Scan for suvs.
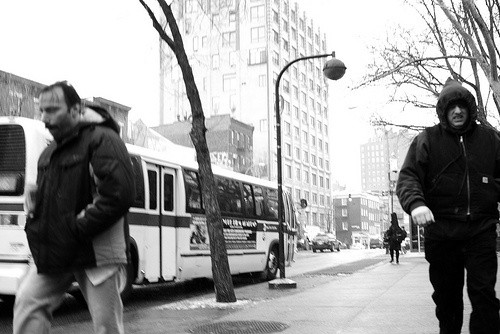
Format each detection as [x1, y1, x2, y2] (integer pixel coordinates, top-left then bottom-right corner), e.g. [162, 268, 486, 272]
[370, 238, 383, 249]
[312, 233, 342, 253]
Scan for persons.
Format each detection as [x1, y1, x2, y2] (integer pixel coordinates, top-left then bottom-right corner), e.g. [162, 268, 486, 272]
[13, 81, 136, 334]
[384, 221, 407, 264]
[396, 78, 500, 334]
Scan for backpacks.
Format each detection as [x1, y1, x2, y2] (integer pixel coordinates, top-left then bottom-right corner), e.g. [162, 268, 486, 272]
[395, 229, 405, 242]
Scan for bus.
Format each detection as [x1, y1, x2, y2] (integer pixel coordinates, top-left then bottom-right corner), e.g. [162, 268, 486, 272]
[0, 116, 308, 306]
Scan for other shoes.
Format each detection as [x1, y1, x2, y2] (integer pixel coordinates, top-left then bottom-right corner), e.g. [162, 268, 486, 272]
[396, 261, 399, 263]
[390, 260, 393, 262]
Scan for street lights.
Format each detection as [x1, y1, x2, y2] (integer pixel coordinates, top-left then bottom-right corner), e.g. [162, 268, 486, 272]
[274, 51, 348, 280]
